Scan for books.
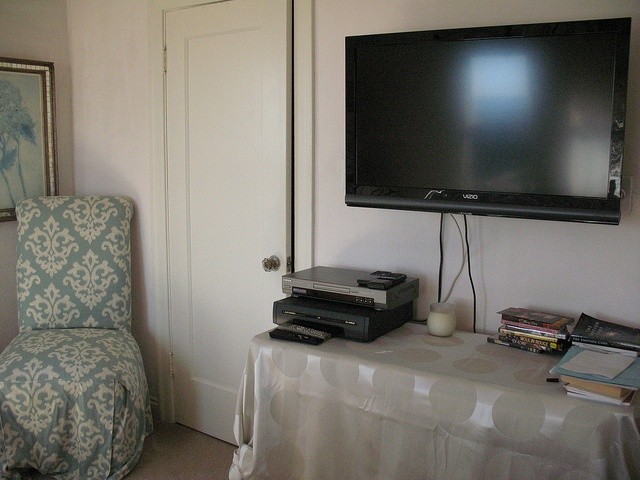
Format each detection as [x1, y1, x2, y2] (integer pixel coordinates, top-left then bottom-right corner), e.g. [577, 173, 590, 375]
[487, 307, 574, 353]
[549, 312, 640, 406]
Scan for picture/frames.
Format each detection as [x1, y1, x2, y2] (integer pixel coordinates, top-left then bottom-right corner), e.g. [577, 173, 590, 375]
[0, 56, 58, 224]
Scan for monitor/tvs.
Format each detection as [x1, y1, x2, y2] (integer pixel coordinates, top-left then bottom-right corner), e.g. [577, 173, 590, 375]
[345, 17, 632, 225]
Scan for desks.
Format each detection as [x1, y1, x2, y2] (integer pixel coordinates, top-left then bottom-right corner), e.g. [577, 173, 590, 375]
[229, 318, 639, 479]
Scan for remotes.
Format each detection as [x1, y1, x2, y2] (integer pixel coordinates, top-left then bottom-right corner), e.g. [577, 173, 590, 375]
[277, 323, 332, 341]
[269, 328, 324, 345]
[356, 271, 391, 286]
[367, 273, 407, 290]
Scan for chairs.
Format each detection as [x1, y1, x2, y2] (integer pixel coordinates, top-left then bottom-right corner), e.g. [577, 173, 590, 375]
[1, 196, 154, 480]
